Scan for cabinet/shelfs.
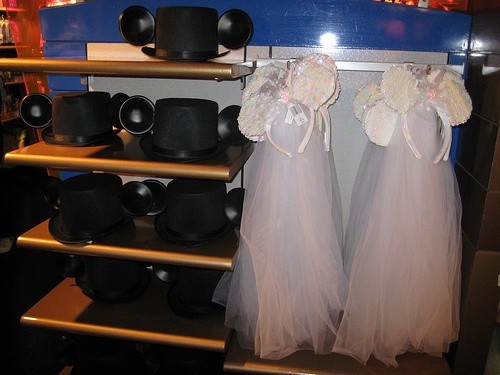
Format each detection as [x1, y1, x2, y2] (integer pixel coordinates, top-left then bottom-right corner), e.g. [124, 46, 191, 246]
[0, 11, 50, 177]
[0, 43, 500, 375]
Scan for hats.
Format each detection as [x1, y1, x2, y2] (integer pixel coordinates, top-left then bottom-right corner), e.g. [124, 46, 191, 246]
[118, 95, 246, 161]
[147, 343, 224, 375]
[59, 253, 156, 305]
[141, 178, 246, 248]
[20, 90, 130, 146]
[151, 262, 230, 321]
[117, 5, 254, 63]
[52, 333, 148, 375]
[40, 172, 152, 244]
